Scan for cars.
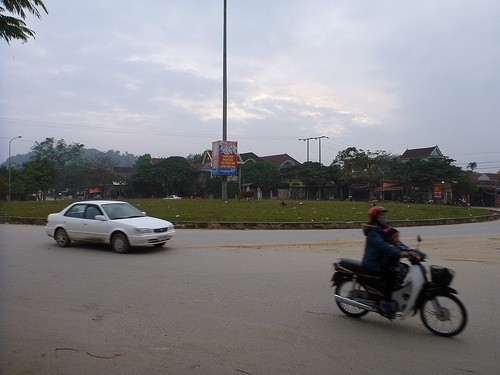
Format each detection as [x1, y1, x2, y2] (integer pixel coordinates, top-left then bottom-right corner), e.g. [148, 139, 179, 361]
[46, 200, 175, 255]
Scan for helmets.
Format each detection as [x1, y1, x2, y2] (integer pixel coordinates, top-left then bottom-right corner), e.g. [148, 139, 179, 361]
[368, 205, 388, 219]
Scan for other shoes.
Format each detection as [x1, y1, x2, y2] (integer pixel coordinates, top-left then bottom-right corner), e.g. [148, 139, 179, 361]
[380, 301, 405, 319]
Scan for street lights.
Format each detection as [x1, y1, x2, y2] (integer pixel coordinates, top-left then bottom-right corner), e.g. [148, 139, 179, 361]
[8, 135, 22, 201]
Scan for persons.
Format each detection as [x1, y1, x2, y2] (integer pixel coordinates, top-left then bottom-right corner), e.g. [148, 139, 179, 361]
[362, 205, 419, 316]
[381, 227, 414, 292]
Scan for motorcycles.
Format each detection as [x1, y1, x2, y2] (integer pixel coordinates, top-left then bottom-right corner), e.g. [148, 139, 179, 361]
[330, 235, 468, 338]
